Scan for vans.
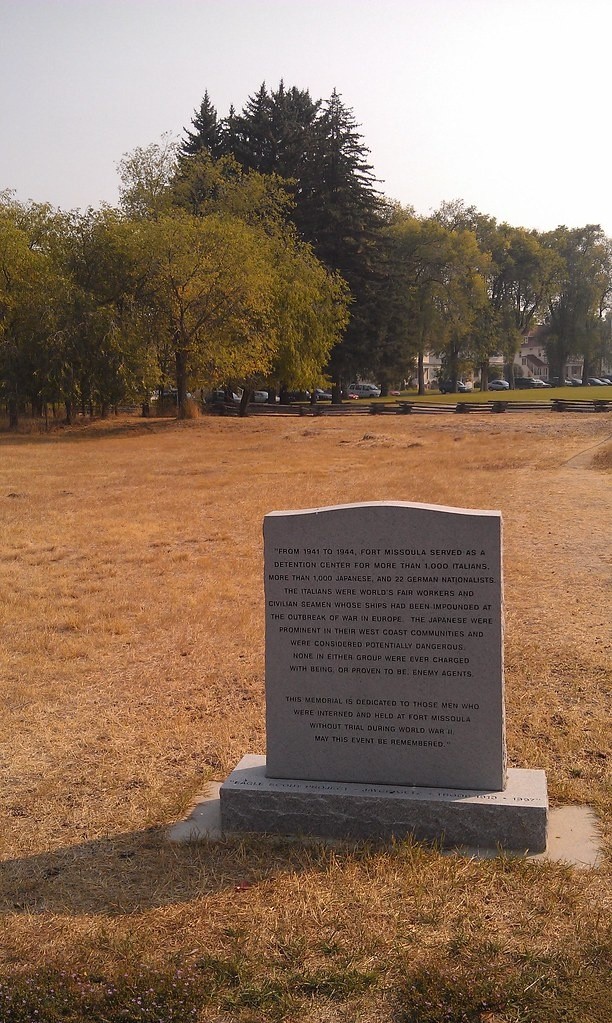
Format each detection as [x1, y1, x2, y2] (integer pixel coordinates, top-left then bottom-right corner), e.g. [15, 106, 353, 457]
[347, 384, 382, 397]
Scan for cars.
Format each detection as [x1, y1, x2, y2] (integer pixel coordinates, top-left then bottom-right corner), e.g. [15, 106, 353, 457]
[513, 377, 543, 389]
[533, 379, 551, 388]
[487, 380, 509, 391]
[376, 383, 401, 396]
[315, 389, 333, 400]
[439, 381, 467, 394]
[598, 375, 612, 386]
[294, 391, 319, 401]
[570, 377, 590, 387]
[205, 390, 241, 404]
[580, 377, 608, 386]
[149, 388, 193, 402]
[553, 377, 573, 386]
[252, 390, 282, 403]
